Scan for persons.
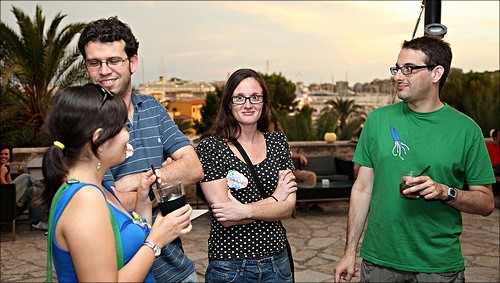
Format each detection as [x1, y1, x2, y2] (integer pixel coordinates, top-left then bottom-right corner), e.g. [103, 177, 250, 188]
[290, 150, 317, 213]
[485, 130, 500, 177]
[196, 69, 298, 283]
[41, 82, 193, 283]
[333, 36, 496, 283]
[0, 142, 49, 235]
[78, 15, 204, 283]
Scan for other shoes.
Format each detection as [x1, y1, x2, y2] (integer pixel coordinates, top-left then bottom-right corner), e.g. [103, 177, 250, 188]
[309, 204, 323, 212]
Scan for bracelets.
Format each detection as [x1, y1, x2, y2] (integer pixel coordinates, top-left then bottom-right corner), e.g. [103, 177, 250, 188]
[270, 196, 279, 202]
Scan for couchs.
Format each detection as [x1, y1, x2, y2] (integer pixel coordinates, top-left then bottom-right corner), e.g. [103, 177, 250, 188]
[293, 156, 354, 181]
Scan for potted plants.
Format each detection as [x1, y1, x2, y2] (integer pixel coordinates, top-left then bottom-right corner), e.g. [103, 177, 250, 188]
[321, 108, 341, 143]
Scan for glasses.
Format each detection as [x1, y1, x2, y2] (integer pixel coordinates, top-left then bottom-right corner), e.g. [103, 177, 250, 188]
[84, 83, 114, 110]
[86, 57, 129, 69]
[231, 96, 264, 105]
[390, 66, 429, 75]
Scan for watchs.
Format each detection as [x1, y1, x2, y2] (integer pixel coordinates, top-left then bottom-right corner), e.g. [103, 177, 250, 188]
[441, 185, 456, 203]
[144, 240, 161, 257]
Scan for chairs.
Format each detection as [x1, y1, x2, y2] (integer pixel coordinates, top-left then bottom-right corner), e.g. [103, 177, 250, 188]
[0, 172, 33, 240]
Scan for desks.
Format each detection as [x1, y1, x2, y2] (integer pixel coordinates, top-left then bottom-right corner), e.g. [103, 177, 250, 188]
[291, 180, 353, 219]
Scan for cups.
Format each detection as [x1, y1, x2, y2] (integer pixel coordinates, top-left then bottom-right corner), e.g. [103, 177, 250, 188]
[398, 171, 421, 200]
[153, 182, 188, 228]
